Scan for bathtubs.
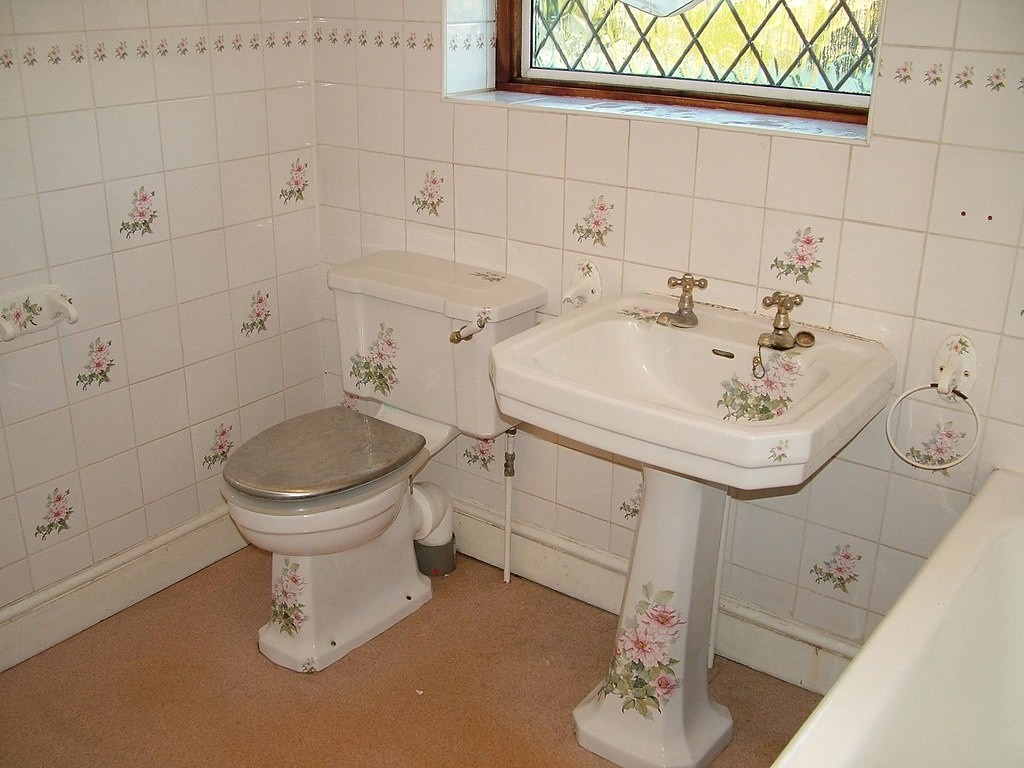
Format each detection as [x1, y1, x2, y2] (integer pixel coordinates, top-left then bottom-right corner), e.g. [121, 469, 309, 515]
[768, 467, 1024, 767]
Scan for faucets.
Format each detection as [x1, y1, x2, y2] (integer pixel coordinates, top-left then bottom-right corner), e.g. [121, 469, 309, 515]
[757, 291, 804, 350]
[656, 272, 708, 328]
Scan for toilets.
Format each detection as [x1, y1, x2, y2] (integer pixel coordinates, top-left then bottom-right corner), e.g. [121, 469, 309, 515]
[217, 249, 548, 673]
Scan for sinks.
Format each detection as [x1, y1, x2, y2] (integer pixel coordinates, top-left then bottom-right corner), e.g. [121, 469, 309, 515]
[488, 291, 898, 491]
